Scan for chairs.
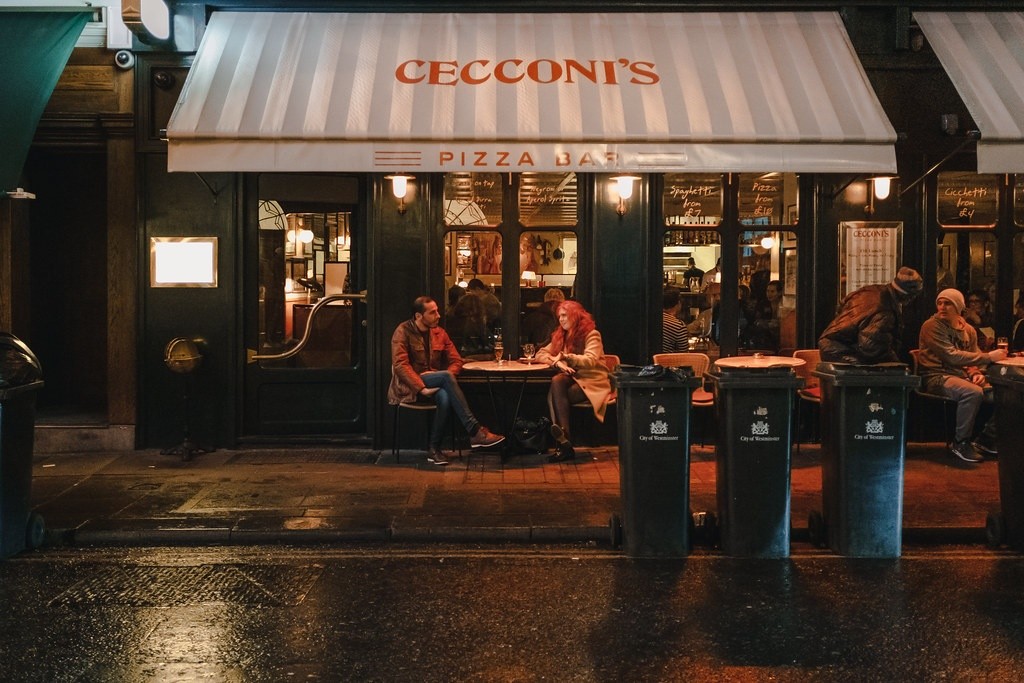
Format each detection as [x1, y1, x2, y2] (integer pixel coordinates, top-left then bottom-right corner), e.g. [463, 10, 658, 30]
[791, 349, 823, 455]
[651, 351, 718, 454]
[570, 351, 620, 456]
[908, 348, 959, 453]
[391, 364, 437, 462]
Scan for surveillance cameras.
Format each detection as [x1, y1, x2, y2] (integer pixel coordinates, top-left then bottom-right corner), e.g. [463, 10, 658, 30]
[113, 49, 135, 70]
[153, 71, 172, 88]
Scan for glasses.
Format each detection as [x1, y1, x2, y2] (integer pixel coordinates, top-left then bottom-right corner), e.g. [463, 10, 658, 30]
[969, 299, 980, 306]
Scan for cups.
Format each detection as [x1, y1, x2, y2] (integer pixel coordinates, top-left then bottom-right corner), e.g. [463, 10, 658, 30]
[997, 336, 1008, 352]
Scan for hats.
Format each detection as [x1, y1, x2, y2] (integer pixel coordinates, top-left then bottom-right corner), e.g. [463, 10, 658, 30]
[891, 266, 924, 293]
[937, 288, 965, 315]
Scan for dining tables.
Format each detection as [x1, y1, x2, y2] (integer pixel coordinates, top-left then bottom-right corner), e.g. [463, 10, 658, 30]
[462, 357, 552, 466]
[993, 354, 1024, 375]
[712, 354, 808, 378]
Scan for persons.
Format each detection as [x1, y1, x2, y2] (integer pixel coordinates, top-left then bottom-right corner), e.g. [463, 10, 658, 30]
[1012, 296, 1024, 352]
[443, 256, 791, 361]
[817, 265, 924, 365]
[962, 288, 997, 347]
[916, 287, 998, 462]
[386, 295, 506, 466]
[535, 301, 612, 463]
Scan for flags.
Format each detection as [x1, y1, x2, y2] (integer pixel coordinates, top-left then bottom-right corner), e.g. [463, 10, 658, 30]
[0, 4, 95, 196]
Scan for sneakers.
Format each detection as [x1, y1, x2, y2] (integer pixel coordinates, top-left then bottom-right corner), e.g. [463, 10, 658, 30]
[974, 432, 998, 454]
[952, 438, 985, 462]
[470, 426, 505, 448]
[427, 448, 448, 464]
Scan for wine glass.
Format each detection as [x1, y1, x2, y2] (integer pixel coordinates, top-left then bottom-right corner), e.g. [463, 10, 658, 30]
[494, 342, 504, 366]
[524, 343, 535, 367]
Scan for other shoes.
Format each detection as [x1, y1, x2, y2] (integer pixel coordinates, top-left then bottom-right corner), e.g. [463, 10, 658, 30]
[545, 448, 575, 462]
[551, 423, 572, 451]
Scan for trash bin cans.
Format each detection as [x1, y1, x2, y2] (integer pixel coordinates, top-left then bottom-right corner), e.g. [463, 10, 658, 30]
[1, 380, 48, 561]
[809, 359, 922, 561]
[609, 365, 695, 560]
[703, 363, 806, 561]
[985, 363, 1024, 552]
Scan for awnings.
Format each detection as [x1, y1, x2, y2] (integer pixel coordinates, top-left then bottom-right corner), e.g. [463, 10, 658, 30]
[163, 10, 899, 177]
[912, 10, 1023, 176]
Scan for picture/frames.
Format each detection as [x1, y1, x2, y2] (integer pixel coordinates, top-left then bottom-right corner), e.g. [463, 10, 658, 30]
[149, 235, 219, 290]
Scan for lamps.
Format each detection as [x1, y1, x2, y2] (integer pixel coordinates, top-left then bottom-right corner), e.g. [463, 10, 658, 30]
[609, 173, 650, 224]
[384, 171, 418, 217]
